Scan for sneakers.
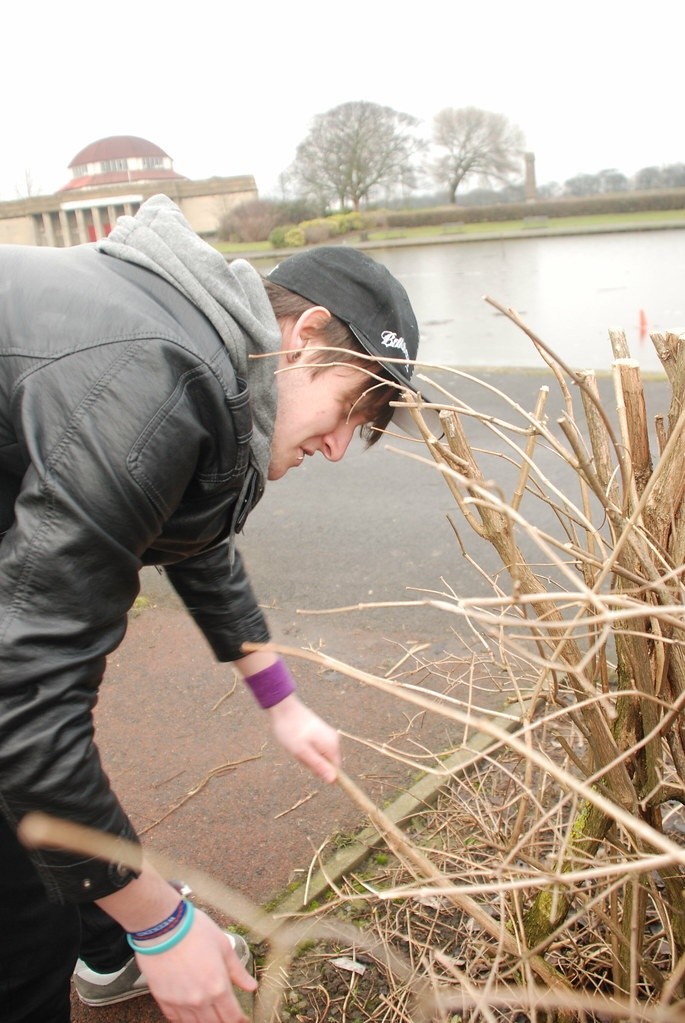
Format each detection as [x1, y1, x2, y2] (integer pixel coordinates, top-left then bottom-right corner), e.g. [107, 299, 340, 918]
[71, 931, 251, 1009]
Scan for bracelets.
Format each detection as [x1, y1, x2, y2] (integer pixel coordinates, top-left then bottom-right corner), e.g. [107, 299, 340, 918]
[243, 663, 295, 709]
[127, 896, 195, 954]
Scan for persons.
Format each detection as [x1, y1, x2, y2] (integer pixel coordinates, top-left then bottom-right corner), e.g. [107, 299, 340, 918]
[0, 190, 450, 1023]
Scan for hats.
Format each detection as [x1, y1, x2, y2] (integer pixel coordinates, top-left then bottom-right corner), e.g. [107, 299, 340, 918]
[269, 246, 422, 394]
